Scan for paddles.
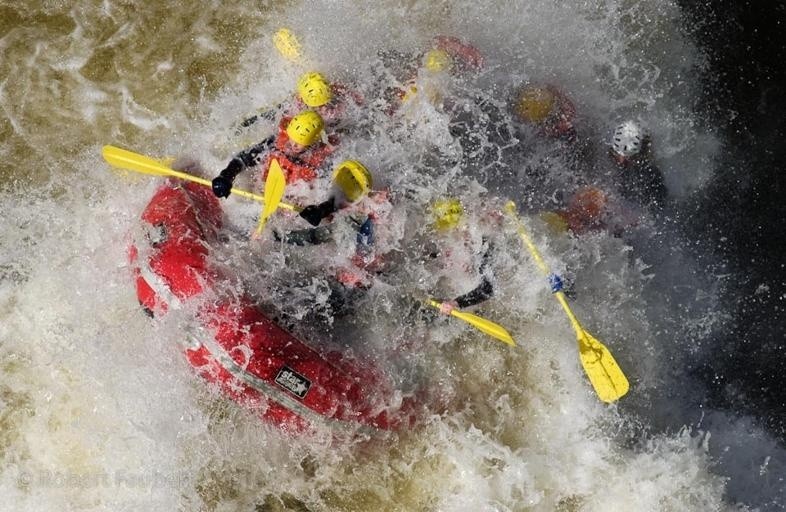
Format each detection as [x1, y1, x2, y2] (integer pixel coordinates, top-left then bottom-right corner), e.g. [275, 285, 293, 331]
[103, 146, 294, 212]
[426, 301, 515, 346]
[506, 202, 629, 402]
[255, 159, 286, 233]
[274, 29, 306, 60]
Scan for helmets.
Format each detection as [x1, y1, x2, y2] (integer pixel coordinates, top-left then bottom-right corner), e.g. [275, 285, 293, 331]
[611, 120, 645, 157]
[295, 72, 333, 107]
[286, 109, 324, 146]
[536, 186, 608, 235]
[402, 49, 451, 119]
[515, 86, 552, 121]
[430, 197, 463, 231]
[332, 160, 372, 201]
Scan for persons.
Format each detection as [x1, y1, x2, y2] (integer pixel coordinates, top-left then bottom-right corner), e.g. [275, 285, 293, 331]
[212, 36, 668, 332]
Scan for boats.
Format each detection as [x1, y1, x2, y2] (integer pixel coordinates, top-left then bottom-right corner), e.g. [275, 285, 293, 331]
[129, 163, 446, 436]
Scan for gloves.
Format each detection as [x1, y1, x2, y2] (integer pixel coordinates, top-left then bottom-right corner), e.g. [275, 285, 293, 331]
[212, 177, 232, 198]
[546, 273, 562, 294]
[299, 206, 321, 225]
